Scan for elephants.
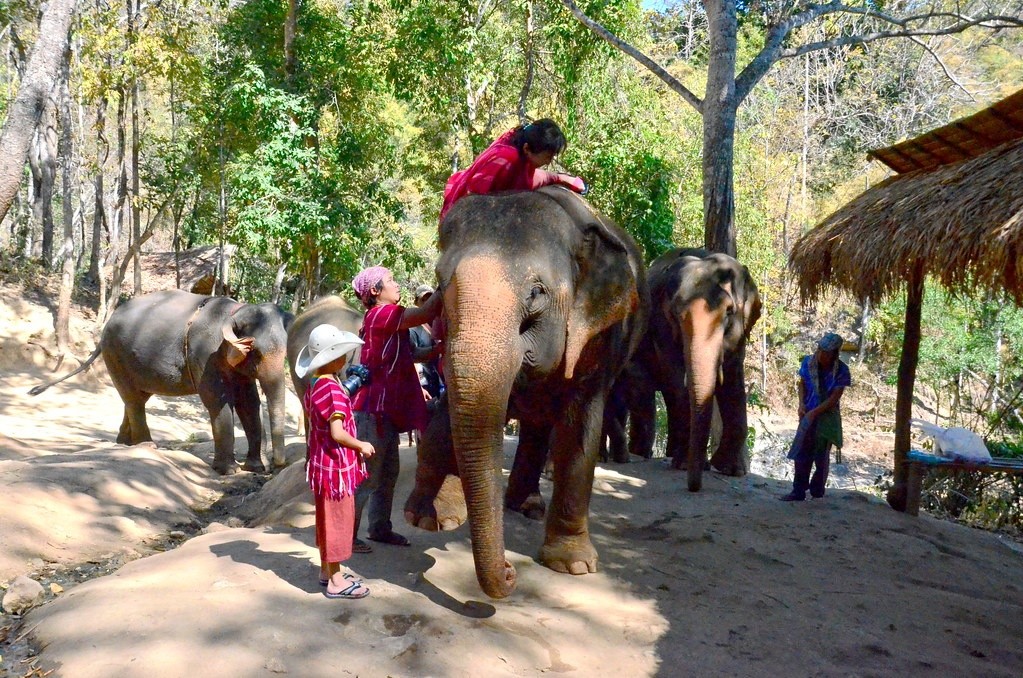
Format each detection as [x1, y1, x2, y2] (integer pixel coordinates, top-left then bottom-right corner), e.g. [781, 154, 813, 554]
[403, 185, 654, 599]
[540, 248, 764, 493]
[287, 293, 366, 437]
[26, 288, 295, 475]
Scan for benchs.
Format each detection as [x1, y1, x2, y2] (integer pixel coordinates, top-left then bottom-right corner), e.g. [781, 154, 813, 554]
[906, 450, 1023, 517]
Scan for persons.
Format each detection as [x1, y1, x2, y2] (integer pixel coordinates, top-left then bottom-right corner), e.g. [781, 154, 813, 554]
[295, 324, 375, 599]
[409, 285, 446, 401]
[779, 334, 850, 501]
[441, 118, 587, 220]
[352, 266, 443, 553]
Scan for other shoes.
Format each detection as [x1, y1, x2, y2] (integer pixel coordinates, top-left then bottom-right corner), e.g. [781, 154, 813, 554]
[812, 491, 824, 501]
[782, 489, 806, 501]
[558, 173, 585, 192]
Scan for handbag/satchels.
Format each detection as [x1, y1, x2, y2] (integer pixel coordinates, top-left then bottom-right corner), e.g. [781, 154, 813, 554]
[909, 417, 993, 466]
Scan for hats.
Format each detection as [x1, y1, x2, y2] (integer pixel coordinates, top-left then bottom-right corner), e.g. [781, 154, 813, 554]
[815, 333, 843, 350]
[414, 284, 435, 298]
[295, 324, 366, 378]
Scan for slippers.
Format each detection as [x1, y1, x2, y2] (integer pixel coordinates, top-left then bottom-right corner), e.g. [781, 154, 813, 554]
[324, 582, 370, 599]
[367, 531, 411, 546]
[351, 537, 373, 552]
[319, 570, 362, 584]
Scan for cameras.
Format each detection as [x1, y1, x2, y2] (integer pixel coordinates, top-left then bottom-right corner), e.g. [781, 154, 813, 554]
[341, 364, 369, 396]
